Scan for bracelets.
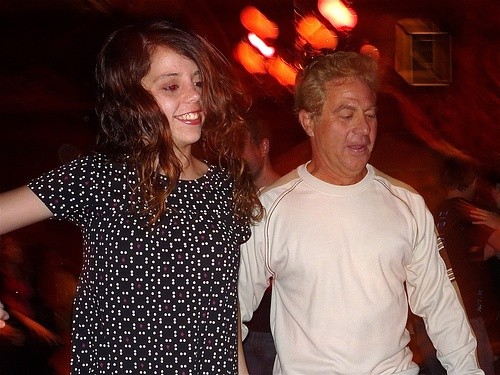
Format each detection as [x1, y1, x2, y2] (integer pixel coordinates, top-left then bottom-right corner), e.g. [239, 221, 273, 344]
[487, 242, 499, 252]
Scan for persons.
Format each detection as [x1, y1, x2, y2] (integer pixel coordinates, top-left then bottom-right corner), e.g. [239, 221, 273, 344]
[0, 20, 267, 374]
[238, 49, 487, 375]
[0, 89, 500, 374]
